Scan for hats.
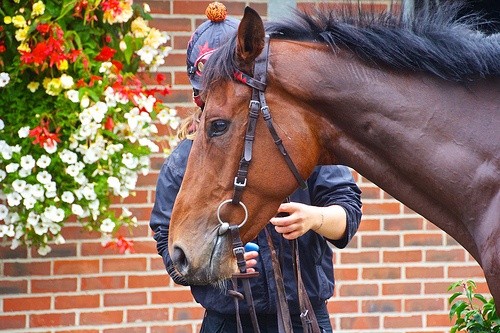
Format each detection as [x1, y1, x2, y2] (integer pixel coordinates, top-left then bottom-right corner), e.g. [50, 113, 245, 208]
[185, 1, 241, 91]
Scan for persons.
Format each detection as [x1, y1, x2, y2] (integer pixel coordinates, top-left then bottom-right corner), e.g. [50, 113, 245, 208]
[149, 1, 362, 333]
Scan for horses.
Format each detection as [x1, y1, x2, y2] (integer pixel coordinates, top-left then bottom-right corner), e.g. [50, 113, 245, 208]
[168, 0, 499, 331]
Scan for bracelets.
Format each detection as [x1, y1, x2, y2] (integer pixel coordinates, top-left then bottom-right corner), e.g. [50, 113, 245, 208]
[312, 206, 324, 232]
